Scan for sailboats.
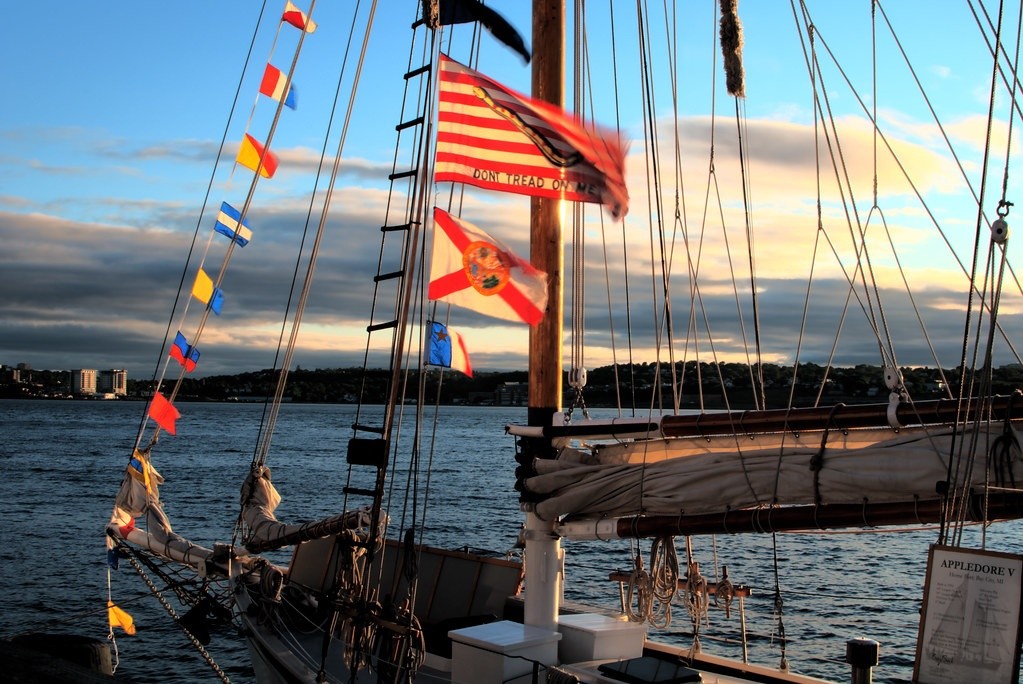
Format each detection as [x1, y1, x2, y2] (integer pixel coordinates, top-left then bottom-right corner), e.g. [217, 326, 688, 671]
[98, 0, 1023, 684]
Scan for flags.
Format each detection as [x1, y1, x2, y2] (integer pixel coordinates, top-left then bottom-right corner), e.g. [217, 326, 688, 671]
[106, 536, 119, 570]
[236, 132, 279, 178]
[125, 450, 152, 496]
[169, 330, 200, 373]
[191, 268, 225, 315]
[281, 1, 318, 33]
[259, 64, 298, 110]
[215, 201, 253, 247]
[423, 320, 473, 379]
[108, 601, 136, 634]
[433, 51, 632, 222]
[148, 391, 181, 436]
[428, 206, 549, 328]
[112, 504, 136, 540]
